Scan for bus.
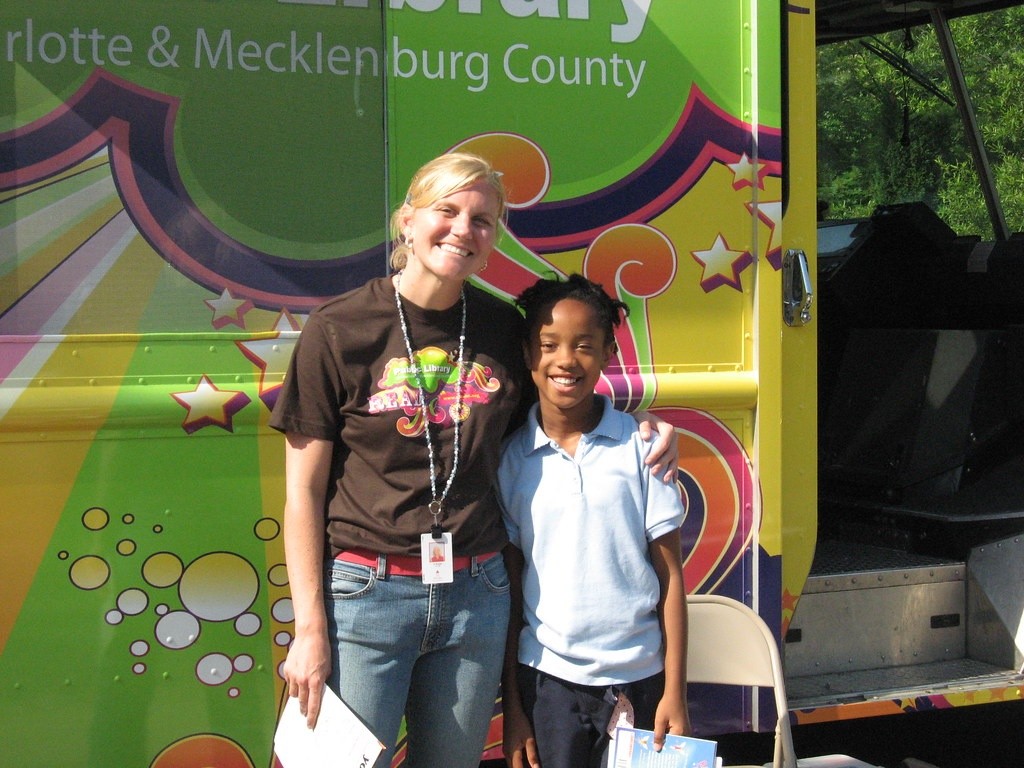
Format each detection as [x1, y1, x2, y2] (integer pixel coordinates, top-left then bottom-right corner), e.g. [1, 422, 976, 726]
[1, 1, 1024, 768]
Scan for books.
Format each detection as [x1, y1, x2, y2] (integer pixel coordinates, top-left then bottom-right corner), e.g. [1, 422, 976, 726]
[273, 681, 387, 768]
[607, 727, 723, 768]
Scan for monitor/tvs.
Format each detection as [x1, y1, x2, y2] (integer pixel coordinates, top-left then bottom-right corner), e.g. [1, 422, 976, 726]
[817, 223, 859, 253]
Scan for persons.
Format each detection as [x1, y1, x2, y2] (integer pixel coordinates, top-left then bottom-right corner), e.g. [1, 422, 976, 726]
[268, 153, 678, 768]
[491, 272, 692, 768]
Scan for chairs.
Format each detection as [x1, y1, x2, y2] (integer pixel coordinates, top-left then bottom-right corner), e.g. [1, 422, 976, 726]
[686, 594, 798, 768]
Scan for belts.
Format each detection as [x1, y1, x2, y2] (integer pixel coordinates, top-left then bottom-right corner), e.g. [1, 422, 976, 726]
[335, 550, 497, 576]
